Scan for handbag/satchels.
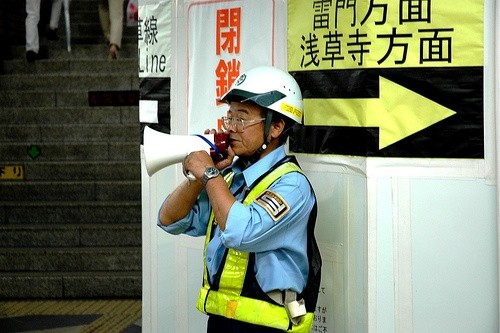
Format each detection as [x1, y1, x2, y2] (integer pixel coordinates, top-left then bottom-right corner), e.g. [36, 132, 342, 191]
[127, 0, 138, 27]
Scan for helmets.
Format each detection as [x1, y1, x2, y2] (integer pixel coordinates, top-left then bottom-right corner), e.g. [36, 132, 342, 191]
[219, 66, 303, 124]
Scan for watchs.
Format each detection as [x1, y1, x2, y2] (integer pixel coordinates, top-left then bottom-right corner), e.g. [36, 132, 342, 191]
[202, 167, 221, 186]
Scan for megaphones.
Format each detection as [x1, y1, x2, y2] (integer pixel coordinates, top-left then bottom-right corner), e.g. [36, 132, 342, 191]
[144, 126, 228, 181]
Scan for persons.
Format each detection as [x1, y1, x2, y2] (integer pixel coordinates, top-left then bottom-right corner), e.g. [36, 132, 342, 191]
[157, 67, 322, 333]
[99, 0, 124, 60]
[25, 0, 63, 63]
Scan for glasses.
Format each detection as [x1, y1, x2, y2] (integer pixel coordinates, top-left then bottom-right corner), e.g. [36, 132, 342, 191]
[221, 116, 267, 133]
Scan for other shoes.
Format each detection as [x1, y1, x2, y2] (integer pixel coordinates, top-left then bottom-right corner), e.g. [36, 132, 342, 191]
[107, 46, 119, 59]
[46, 28, 59, 41]
[25, 50, 39, 63]
